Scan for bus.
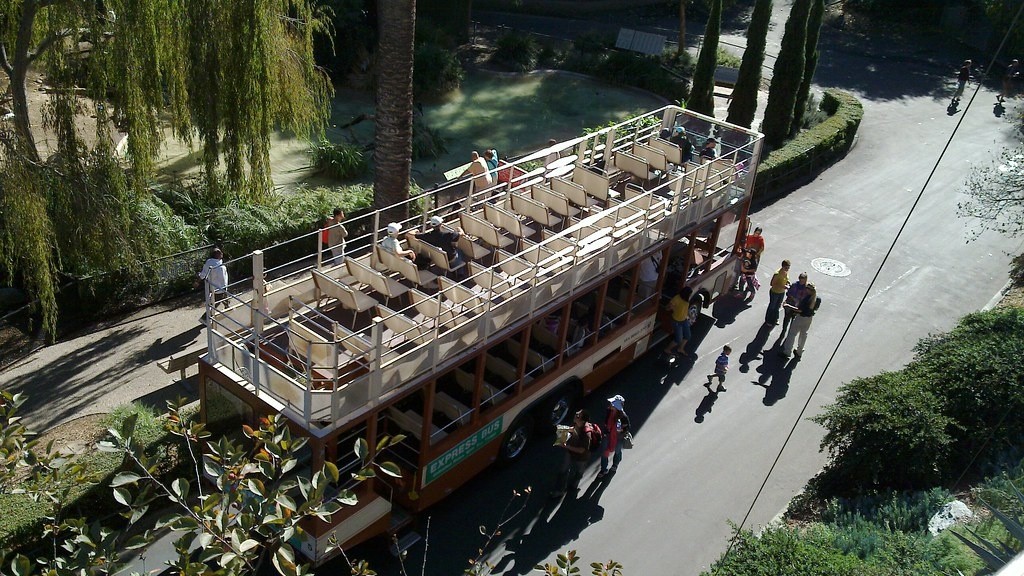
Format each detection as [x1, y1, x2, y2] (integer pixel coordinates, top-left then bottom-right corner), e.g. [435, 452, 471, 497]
[198, 104, 766, 572]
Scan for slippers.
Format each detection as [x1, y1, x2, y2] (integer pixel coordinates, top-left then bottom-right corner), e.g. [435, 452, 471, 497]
[664, 348, 689, 357]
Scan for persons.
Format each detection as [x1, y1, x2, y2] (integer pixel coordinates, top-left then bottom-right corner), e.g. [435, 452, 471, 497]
[735, 227, 765, 300]
[597, 394, 630, 478]
[572, 319, 590, 351]
[551, 409, 591, 505]
[545, 138, 561, 170]
[262, 268, 272, 318]
[996, 59, 1019, 102]
[638, 249, 663, 301]
[659, 127, 693, 174]
[707, 345, 731, 392]
[405, 215, 467, 279]
[776, 273, 817, 359]
[951, 59, 973, 102]
[379, 222, 427, 269]
[765, 260, 791, 327]
[328, 207, 348, 266]
[698, 137, 733, 163]
[198, 248, 230, 327]
[458, 149, 498, 210]
[663, 286, 693, 359]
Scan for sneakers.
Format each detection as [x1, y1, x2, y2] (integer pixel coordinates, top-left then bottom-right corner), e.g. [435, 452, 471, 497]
[597, 464, 617, 478]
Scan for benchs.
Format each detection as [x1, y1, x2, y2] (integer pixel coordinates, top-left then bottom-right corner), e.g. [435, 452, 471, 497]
[388, 276, 651, 447]
[284, 162, 674, 382]
[613, 137, 739, 200]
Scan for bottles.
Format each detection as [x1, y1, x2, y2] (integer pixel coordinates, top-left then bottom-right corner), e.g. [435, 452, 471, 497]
[616, 419, 621, 430]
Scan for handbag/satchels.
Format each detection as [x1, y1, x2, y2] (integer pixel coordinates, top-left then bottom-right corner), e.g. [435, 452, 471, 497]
[322, 224, 328, 244]
[622, 430, 634, 449]
[814, 297, 821, 311]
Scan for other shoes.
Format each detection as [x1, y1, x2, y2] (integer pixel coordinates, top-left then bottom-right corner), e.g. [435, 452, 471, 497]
[750, 292, 755, 299]
[717, 385, 726, 391]
[198, 318, 207, 327]
[794, 348, 802, 358]
[707, 375, 712, 384]
[767, 318, 780, 326]
[736, 294, 744, 298]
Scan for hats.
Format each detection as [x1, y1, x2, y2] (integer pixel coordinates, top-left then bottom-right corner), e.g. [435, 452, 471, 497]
[387, 222, 402, 234]
[660, 130, 672, 139]
[607, 395, 625, 411]
[706, 138, 719, 143]
[676, 127, 685, 133]
[805, 282, 817, 292]
[575, 409, 591, 420]
[745, 248, 757, 256]
[431, 216, 444, 226]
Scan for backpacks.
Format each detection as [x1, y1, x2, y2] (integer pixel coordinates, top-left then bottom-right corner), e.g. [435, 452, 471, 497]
[583, 422, 607, 462]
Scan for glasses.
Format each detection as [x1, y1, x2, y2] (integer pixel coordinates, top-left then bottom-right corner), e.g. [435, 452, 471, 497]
[676, 131, 678, 132]
